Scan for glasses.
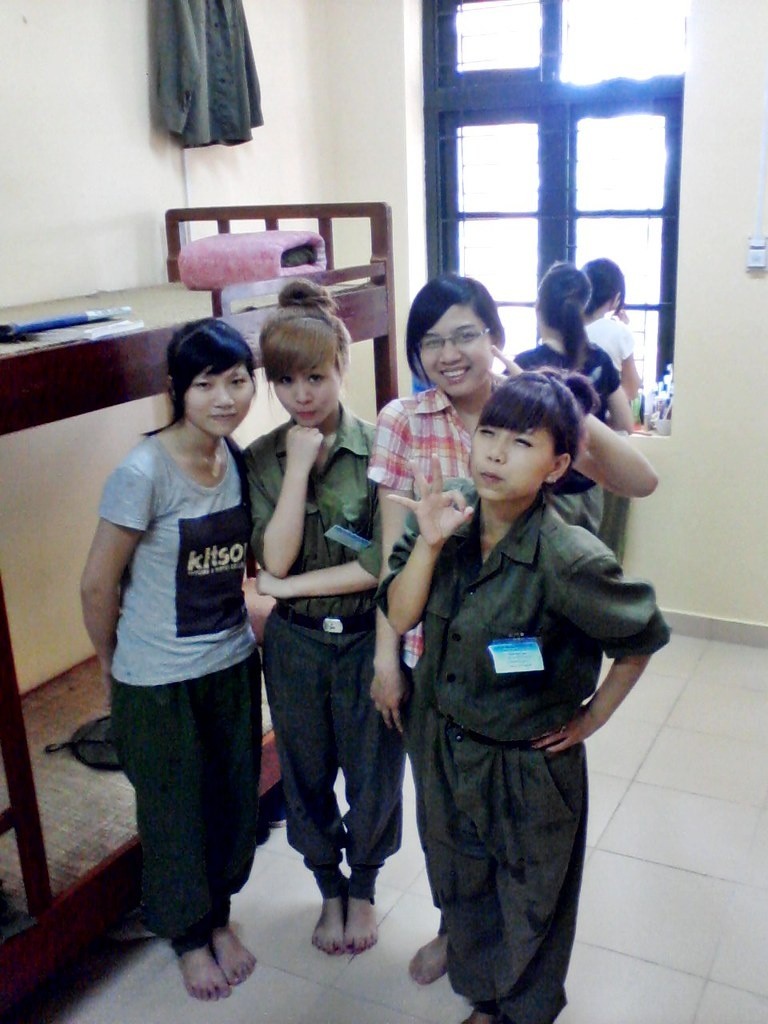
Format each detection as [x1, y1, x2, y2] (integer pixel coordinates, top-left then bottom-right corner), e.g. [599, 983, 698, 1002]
[419, 326, 490, 350]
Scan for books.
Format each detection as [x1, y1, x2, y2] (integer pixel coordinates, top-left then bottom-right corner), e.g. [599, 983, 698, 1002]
[0, 308, 143, 343]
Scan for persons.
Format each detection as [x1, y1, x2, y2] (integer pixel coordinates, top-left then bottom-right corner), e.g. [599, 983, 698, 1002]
[503, 256, 641, 568]
[79, 317, 264, 1000]
[239, 279, 406, 954]
[375, 370, 672, 1023]
[369, 276, 658, 983]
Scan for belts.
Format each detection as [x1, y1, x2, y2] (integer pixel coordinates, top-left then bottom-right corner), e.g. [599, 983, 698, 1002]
[276, 603, 377, 633]
[439, 709, 539, 751]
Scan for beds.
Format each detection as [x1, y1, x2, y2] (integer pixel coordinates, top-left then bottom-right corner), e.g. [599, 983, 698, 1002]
[0, 199, 398, 1024]
